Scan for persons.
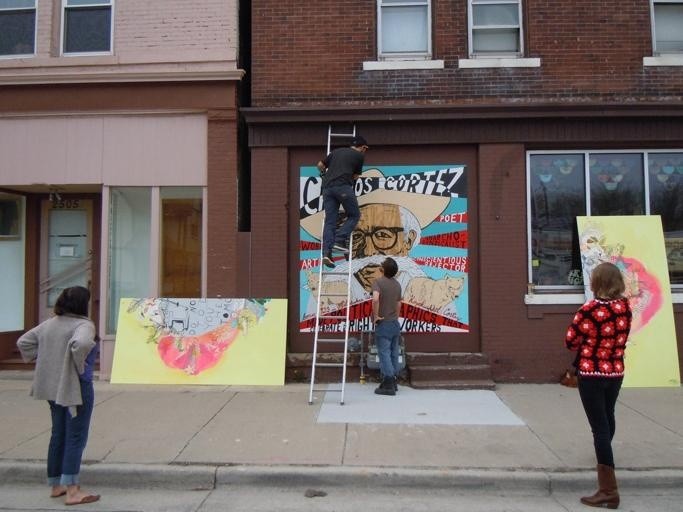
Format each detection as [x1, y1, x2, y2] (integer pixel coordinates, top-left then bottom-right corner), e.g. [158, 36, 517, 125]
[369, 255, 404, 396]
[15, 285, 102, 506]
[315, 134, 370, 269]
[338, 201, 455, 320]
[564, 262, 634, 511]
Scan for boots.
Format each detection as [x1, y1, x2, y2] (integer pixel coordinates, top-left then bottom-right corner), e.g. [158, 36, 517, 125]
[375, 380, 398, 395]
[581, 464, 620, 509]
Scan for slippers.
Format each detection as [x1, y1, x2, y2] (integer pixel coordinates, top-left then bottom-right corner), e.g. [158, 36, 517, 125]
[64, 494, 100, 505]
[51, 483, 81, 498]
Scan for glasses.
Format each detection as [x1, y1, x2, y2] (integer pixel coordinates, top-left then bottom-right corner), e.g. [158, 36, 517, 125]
[344, 226, 405, 251]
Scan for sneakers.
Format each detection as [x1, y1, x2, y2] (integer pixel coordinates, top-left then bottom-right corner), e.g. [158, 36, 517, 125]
[323, 256, 335, 268]
[333, 244, 348, 251]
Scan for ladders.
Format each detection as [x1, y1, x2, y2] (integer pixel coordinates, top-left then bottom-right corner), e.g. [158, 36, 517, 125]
[308, 125, 357, 405]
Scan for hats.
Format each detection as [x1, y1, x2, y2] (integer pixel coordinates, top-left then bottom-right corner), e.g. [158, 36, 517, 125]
[352, 136, 371, 151]
